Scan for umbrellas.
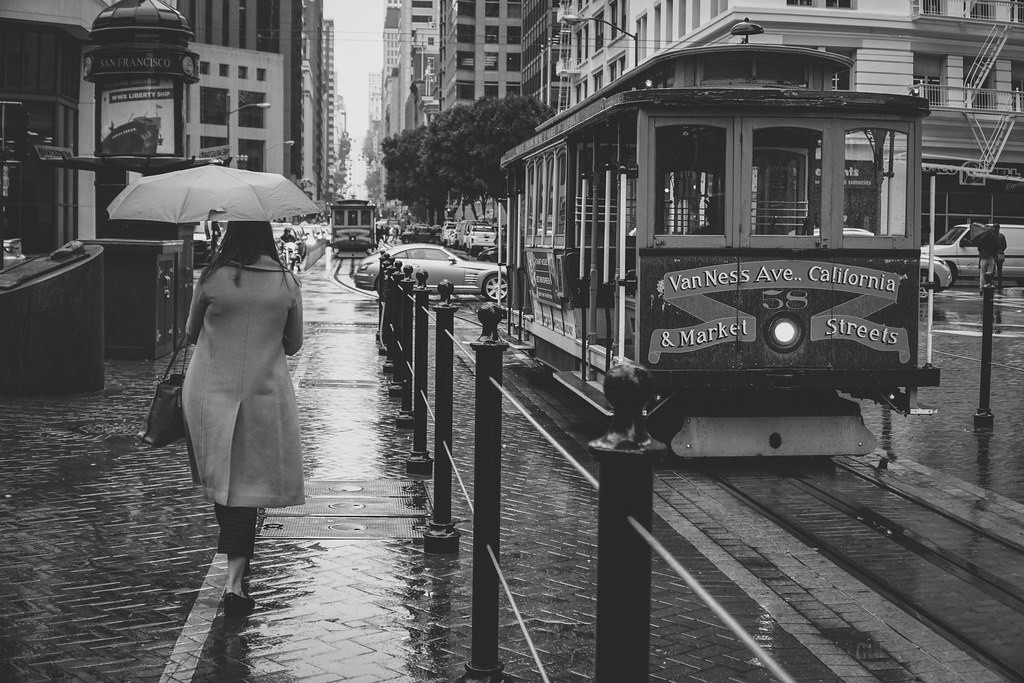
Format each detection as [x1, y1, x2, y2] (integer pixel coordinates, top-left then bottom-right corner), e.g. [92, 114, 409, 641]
[106, 166, 325, 273]
[971, 222, 1000, 257]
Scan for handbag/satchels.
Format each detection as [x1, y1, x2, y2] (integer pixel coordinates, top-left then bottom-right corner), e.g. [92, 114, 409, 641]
[136, 333, 190, 448]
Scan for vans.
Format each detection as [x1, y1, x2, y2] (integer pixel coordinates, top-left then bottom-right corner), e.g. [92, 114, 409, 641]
[917, 222, 1024, 286]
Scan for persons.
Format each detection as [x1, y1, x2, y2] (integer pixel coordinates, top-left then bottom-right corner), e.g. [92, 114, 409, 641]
[375, 223, 402, 245]
[980, 248, 997, 296]
[690, 194, 725, 236]
[278, 226, 302, 274]
[184, 223, 307, 616]
[990, 224, 1009, 295]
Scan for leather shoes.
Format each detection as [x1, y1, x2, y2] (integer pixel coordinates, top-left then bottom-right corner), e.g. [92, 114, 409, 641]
[224, 566, 256, 613]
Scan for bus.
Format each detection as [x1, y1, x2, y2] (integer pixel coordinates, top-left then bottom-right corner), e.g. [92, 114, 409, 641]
[329, 196, 377, 253]
[494, 37, 945, 466]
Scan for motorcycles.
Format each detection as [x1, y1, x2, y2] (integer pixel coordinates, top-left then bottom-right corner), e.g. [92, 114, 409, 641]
[279, 233, 310, 272]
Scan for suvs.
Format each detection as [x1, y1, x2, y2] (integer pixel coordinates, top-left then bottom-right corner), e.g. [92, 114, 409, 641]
[806, 224, 956, 300]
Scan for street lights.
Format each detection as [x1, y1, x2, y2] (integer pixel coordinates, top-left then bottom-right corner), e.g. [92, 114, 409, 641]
[263, 138, 296, 172]
[562, 15, 640, 69]
[224, 101, 272, 169]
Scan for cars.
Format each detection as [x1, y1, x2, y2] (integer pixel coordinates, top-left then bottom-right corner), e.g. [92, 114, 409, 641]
[193, 219, 223, 259]
[375, 218, 510, 264]
[268, 219, 331, 249]
[354, 241, 510, 303]
